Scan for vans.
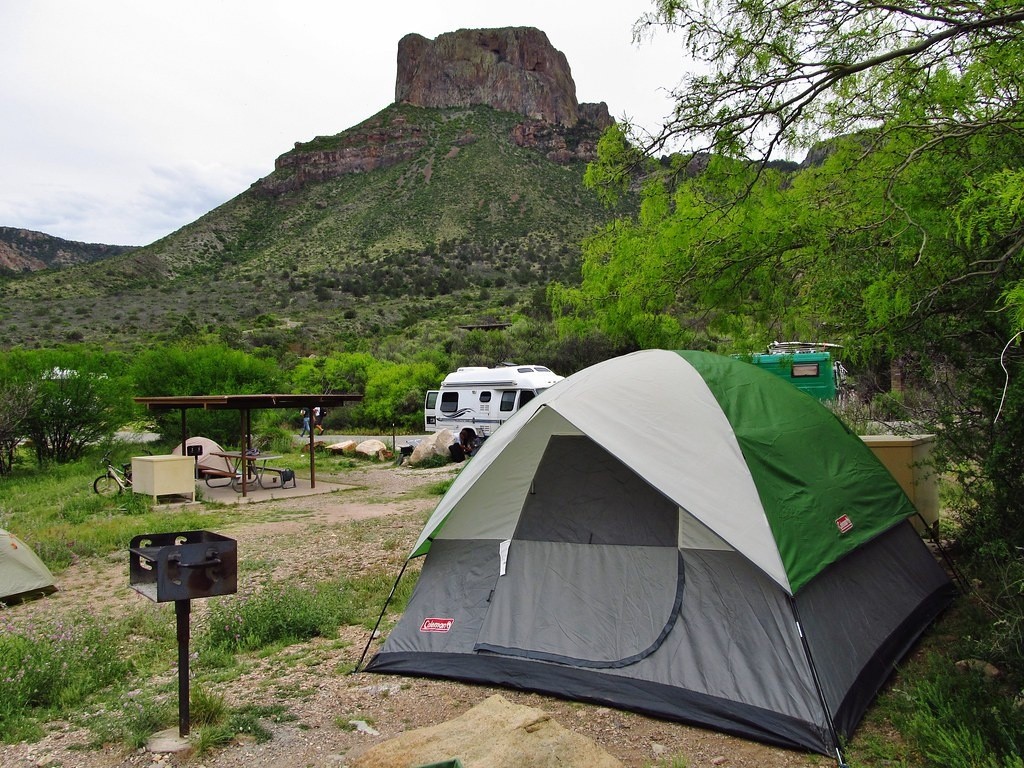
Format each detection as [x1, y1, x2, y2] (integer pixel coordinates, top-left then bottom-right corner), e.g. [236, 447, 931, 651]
[731, 354, 835, 403]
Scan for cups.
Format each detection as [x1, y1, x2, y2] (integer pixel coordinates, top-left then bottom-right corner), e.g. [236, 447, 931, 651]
[273, 477, 276, 483]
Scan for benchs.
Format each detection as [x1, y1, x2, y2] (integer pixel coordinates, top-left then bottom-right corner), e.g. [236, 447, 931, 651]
[246, 465, 290, 486]
[202, 469, 242, 491]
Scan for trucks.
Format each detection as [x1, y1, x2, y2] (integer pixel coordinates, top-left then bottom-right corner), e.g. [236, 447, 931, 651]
[425, 363, 564, 452]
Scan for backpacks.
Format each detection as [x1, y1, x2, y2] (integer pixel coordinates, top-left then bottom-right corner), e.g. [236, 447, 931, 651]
[320, 406, 327, 418]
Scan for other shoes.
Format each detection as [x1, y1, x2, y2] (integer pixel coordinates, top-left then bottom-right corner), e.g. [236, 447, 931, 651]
[320, 429, 324, 434]
[317, 433, 322, 436]
[298, 434, 303, 437]
[307, 435, 311, 438]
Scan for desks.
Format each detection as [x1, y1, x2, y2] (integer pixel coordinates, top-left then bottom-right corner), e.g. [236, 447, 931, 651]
[132, 454, 196, 504]
[859, 434, 940, 544]
[210, 451, 283, 490]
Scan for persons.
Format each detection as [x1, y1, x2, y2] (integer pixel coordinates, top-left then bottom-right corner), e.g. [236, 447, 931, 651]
[299, 408, 310, 438]
[313, 407, 324, 436]
[454, 430, 480, 452]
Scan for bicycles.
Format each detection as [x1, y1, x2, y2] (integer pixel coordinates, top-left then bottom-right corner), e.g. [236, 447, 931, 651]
[93, 450, 132, 497]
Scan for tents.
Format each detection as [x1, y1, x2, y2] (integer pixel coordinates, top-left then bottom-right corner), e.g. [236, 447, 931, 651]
[353, 349, 971, 767]
[171, 436, 234, 473]
[0, 528, 59, 606]
